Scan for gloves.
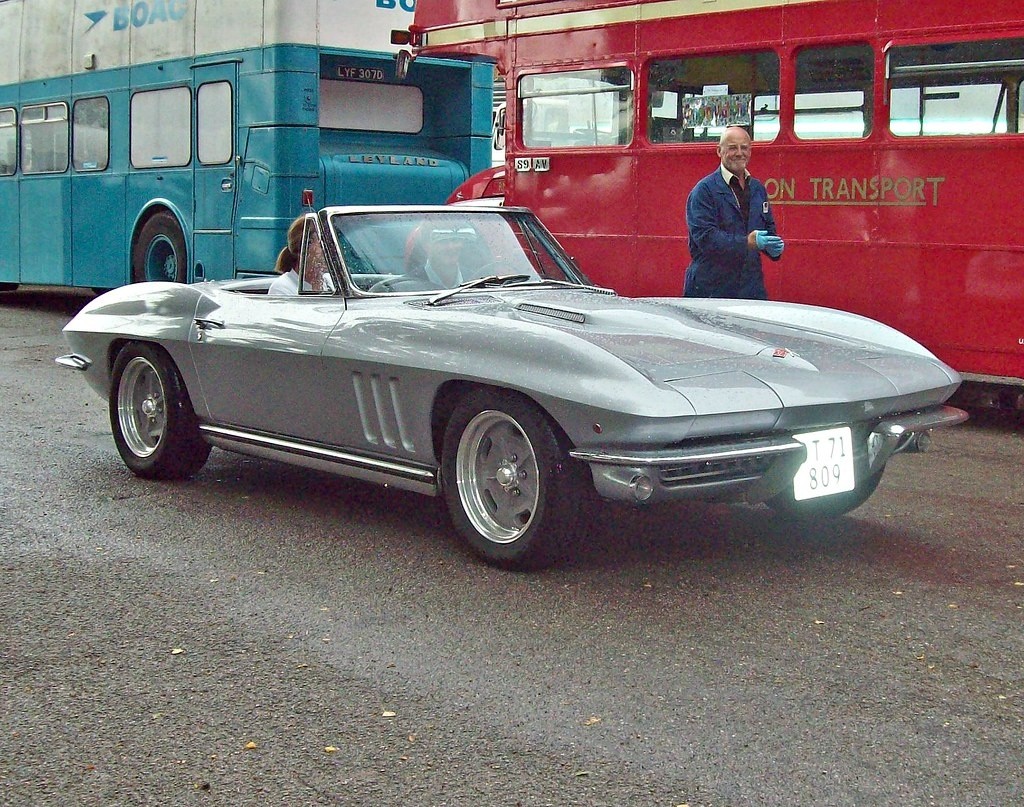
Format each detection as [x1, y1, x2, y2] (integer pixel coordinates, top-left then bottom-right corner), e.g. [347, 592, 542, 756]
[755, 230, 782, 250]
[765, 236, 784, 257]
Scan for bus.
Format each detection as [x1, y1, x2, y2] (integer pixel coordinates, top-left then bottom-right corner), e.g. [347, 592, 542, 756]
[0, 1, 492, 304]
[386, 0, 1024, 432]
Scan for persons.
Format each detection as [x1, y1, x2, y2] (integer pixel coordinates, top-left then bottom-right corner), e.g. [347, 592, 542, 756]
[402, 220, 480, 290]
[684, 127, 784, 300]
[267, 214, 337, 295]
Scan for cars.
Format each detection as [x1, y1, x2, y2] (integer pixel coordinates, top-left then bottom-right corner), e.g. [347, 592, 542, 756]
[54, 207, 968, 570]
